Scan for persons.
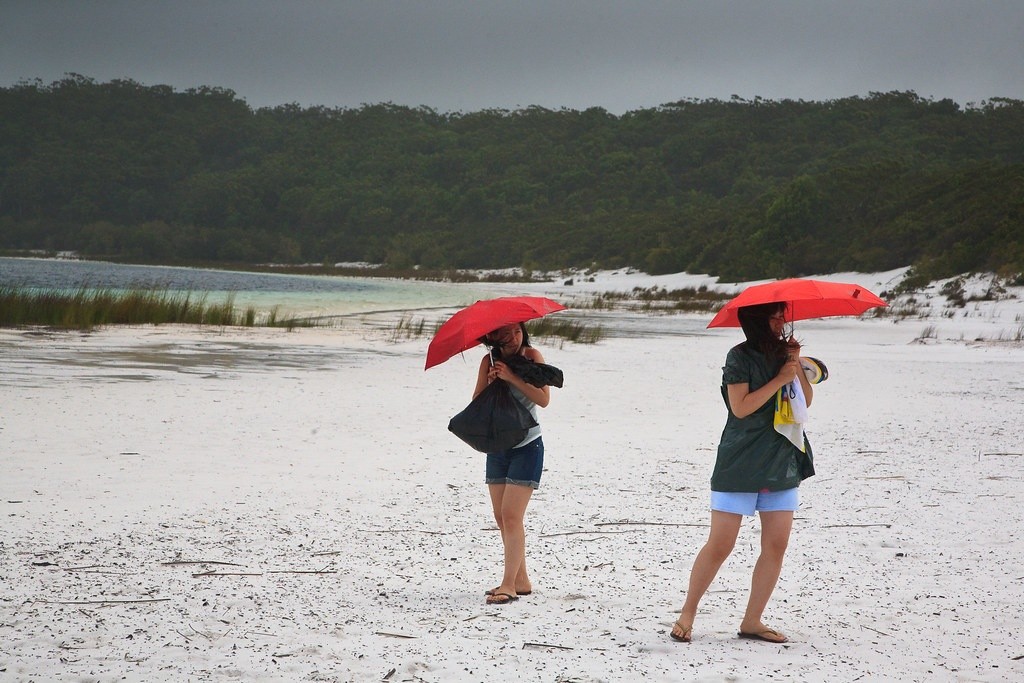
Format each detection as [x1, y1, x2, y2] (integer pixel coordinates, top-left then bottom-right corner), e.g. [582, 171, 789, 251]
[472, 321, 550, 604]
[669, 302, 816, 643]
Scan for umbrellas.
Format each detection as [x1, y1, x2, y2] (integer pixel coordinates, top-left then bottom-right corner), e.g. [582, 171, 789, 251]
[424, 296, 568, 372]
[707, 277, 891, 337]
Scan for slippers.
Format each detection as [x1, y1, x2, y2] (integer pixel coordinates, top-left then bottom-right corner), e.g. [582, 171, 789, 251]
[670, 620, 692, 642]
[485, 587, 532, 595]
[738, 629, 788, 643]
[487, 592, 519, 604]
